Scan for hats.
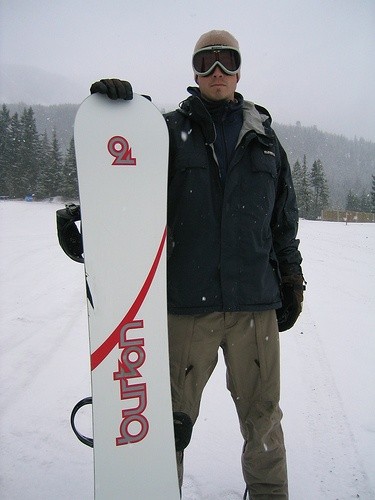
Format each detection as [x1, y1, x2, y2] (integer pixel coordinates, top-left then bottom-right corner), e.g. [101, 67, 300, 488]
[194, 30, 239, 51]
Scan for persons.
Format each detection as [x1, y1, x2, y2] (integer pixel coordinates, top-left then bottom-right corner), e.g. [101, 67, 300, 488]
[90, 30, 307, 500]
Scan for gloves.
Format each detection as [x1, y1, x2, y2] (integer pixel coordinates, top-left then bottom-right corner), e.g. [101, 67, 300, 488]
[91, 79, 152, 102]
[277, 276, 308, 331]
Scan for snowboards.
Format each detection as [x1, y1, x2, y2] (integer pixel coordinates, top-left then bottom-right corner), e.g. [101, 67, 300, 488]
[73, 91, 182, 500]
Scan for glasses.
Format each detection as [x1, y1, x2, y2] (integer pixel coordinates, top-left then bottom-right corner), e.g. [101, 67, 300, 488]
[192, 46, 241, 76]
